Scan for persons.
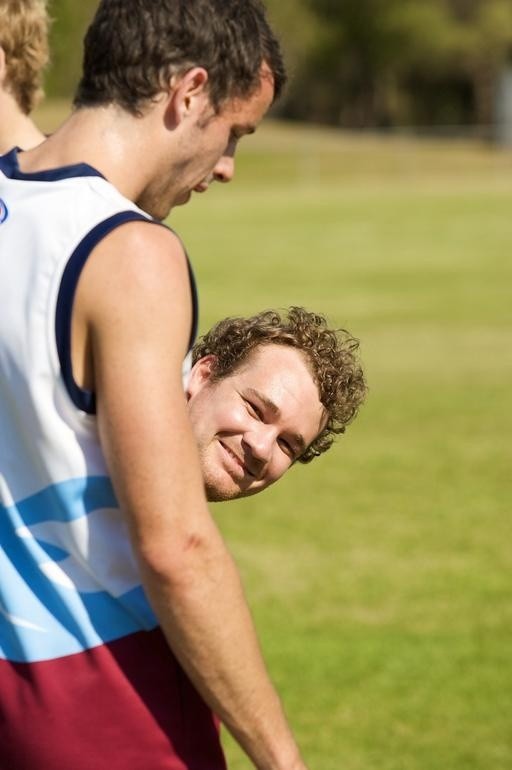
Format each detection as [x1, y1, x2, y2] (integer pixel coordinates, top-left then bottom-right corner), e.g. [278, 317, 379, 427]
[1, 0, 56, 158]
[183, 305, 368, 503]
[0, 0, 311, 769]
